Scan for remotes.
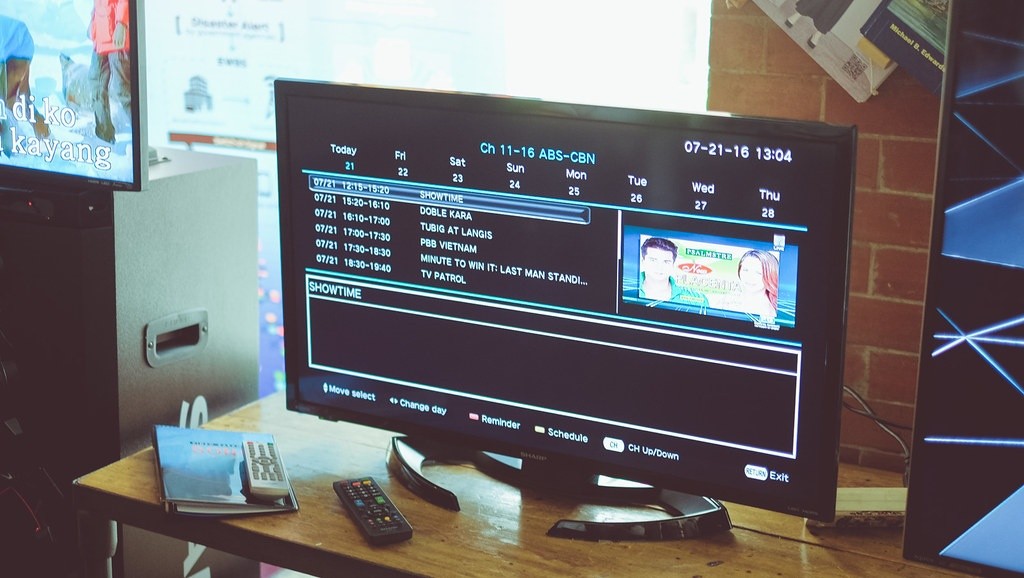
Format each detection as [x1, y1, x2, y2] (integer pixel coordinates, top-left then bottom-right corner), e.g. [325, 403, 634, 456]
[332, 476, 415, 546]
[240, 440, 290, 504]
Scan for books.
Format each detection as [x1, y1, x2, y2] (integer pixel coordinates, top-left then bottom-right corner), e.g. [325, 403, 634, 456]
[151, 423, 298, 516]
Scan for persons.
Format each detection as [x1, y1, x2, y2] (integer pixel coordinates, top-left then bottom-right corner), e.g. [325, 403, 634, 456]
[639, 237, 711, 308]
[86, 1, 130, 145]
[0, 13, 58, 149]
[736, 249, 780, 316]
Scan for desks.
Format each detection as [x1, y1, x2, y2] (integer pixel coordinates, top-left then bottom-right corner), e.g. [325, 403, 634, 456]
[67, 393, 1009, 576]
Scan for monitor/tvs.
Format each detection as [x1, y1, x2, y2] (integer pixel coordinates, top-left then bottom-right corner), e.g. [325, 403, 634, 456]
[273, 77, 857, 542]
[0, 0, 148, 192]
